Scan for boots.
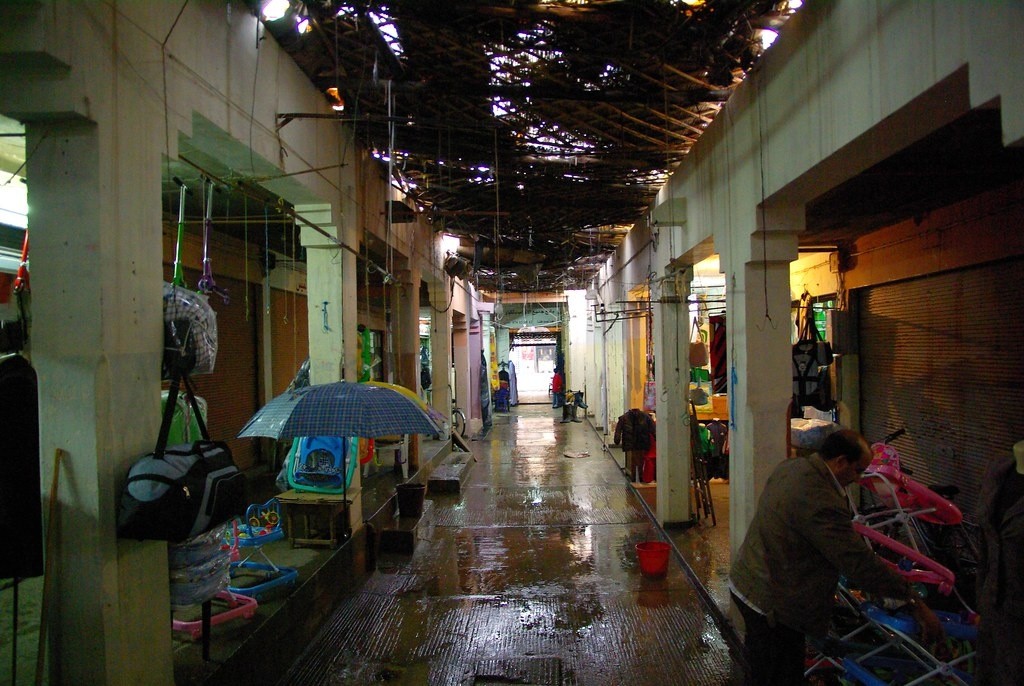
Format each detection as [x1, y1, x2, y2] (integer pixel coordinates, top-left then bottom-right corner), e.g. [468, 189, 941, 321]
[560, 405, 582, 423]
[575, 391, 587, 408]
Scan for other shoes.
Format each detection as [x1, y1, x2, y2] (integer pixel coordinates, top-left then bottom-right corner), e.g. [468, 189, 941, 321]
[552, 404, 559, 407]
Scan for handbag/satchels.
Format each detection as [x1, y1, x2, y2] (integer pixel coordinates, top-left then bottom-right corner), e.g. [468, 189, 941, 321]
[817, 341, 833, 364]
[690, 317, 707, 367]
[162, 281, 216, 380]
[118, 379, 250, 543]
[690, 388, 708, 405]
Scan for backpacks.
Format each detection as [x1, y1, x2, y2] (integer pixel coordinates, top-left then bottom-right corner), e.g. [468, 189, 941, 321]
[422, 349, 429, 365]
[421, 368, 432, 389]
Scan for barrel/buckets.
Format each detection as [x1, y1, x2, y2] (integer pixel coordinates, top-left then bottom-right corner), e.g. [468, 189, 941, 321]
[635, 529, 672, 577]
[397, 484, 426, 516]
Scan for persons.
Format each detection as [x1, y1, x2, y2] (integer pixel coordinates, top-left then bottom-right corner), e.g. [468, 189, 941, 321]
[729, 428, 950, 686]
[552, 367, 564, 408]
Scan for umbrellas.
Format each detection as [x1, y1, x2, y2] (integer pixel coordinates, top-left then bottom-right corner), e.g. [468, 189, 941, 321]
[234, 378, 443, 541]
[357, 374, 451, 468]
